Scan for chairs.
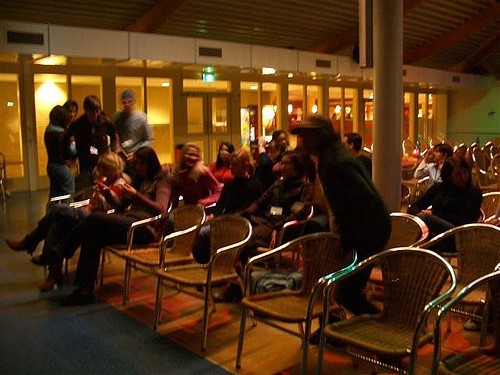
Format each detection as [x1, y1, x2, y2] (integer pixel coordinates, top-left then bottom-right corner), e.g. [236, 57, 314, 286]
[42, 138, 500, 375]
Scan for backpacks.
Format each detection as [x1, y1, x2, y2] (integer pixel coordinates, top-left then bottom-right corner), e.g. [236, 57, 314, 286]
[252, 269, 300, 318]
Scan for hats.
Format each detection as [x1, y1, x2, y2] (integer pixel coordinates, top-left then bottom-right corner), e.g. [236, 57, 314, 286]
[290, 115, 333, 133]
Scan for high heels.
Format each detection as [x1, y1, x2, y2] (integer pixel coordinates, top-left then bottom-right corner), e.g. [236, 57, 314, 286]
[6, 234, 35, 253]
[39, 272, 63, 291]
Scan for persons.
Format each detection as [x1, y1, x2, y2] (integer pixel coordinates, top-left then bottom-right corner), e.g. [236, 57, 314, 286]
[248, 138, 271, 163]
[110, 88, 154, 151]
[165, 144, 223, 207]
[63, 98, 78, 124]
[208, 142, 241, 184]
[288, 114, 391, 320]
[194, 152, 318, 267]
[272, 129, 292, 151]
[214, 149, 262, 218]
[253, 138, 288, 190]
[343, 133, 372, 179]
[44, 104, 78, 210]
[413, 144, 453, 185]
[6, 151, 120, 291]
[30, 148, 173, 306]
[406, 155, 483, 252]
[67, 94, 117, 204]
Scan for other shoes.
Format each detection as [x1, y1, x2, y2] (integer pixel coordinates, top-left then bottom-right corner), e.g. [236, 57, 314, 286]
[464, 313, 483, 330]
[212, 282, 242, 302]
[32, 248, 59, 264]
[310, 326, 343, 345]
[64, 289, 93, 305]
